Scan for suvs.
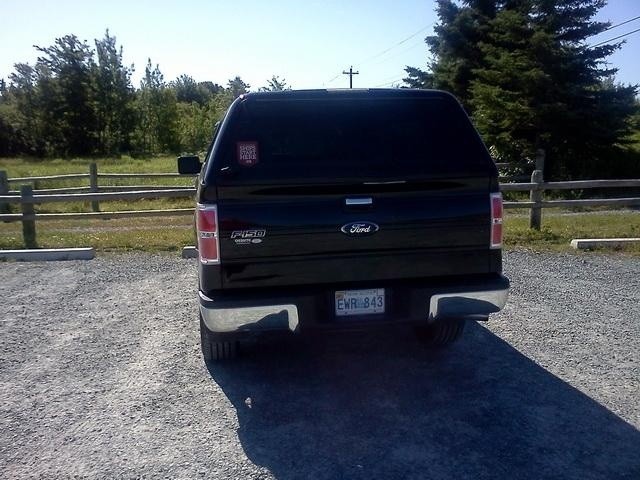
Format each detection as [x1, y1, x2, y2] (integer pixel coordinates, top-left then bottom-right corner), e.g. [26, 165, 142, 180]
[176, 86, 511, 364]
